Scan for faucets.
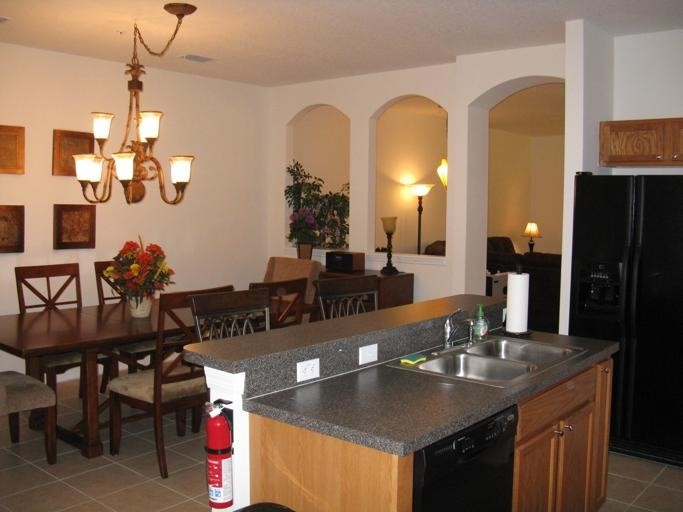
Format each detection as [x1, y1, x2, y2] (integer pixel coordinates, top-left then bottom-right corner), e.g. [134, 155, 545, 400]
[443, 309, 474, 349]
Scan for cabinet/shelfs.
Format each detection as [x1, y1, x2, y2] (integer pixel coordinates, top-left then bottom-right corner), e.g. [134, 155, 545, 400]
[599, 117, 683, 167]
[569, 171, 683, 466]
[512, 357, 614, 512]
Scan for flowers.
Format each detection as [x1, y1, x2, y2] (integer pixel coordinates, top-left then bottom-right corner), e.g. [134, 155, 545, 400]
[104, 236, 176, 302]
[284, 157, 350, 250]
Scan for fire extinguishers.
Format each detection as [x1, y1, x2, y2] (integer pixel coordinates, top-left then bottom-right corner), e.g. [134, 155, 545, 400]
[206, 400, 234, 508]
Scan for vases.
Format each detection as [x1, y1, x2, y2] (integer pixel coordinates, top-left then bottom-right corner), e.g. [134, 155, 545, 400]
[297, 243, 313, 260]
[128, 294, 153, 319]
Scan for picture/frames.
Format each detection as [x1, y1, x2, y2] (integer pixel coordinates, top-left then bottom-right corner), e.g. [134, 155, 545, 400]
[0, 125, 26, 176]
[73, 2, 195, 204]
[53, 204, 96, 249]
[0, 205, 25, 253]
[52, 129, 95, 176]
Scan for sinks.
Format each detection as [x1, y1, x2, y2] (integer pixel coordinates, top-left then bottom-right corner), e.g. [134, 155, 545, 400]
[386, 351, 537, 389]
[464, 334, 590, 366]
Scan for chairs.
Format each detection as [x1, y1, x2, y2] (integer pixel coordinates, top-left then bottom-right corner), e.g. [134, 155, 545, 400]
[14, 263, 84, 400]
[249, 278, 307, 333]
[188, 289, 270, 342]
[1, 370, 57, 464]
[108, 286, 234, 477]
[96, 261, 171, 394]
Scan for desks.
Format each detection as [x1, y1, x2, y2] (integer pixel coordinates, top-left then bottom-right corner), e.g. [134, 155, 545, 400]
[2, 299, 319, 460]
[310, 265, 414, 321]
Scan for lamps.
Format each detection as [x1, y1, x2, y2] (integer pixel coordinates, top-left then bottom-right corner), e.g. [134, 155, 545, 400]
[380, 217, 398, 276]
[520, 221, 543, 252]
[409, 182, 435, 254]
[436, 158, 447, 192]
